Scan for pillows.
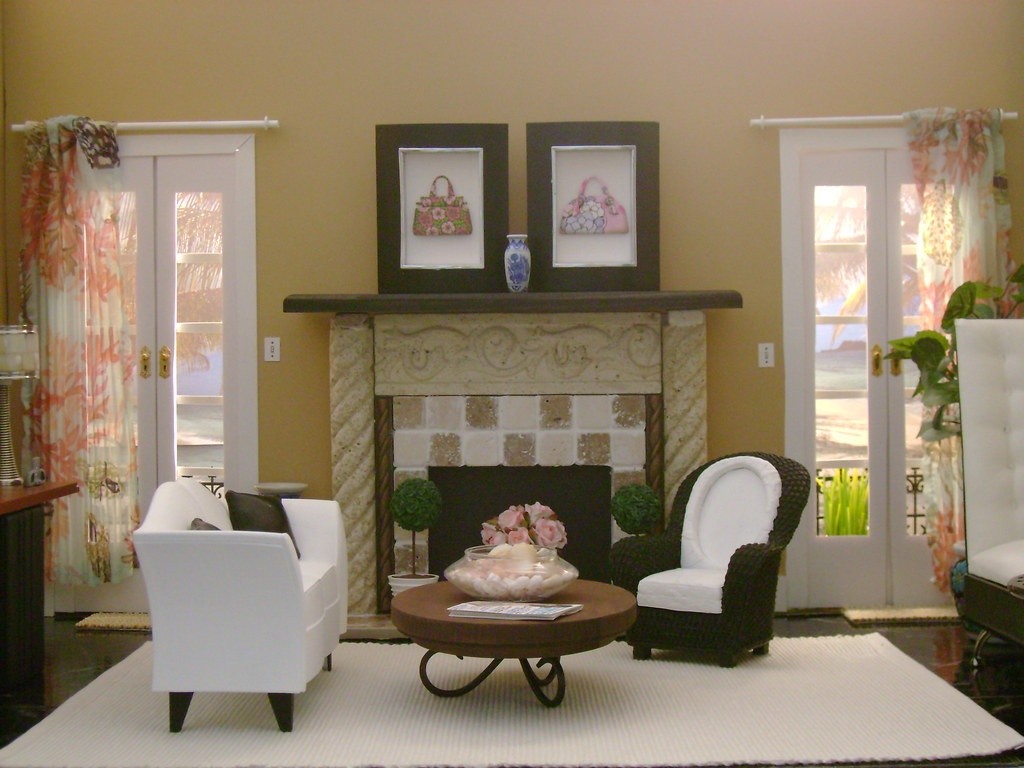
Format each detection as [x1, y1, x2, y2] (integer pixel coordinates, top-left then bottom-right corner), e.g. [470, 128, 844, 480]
[226, 490, 302, 560]
[192, 518, 219, 531]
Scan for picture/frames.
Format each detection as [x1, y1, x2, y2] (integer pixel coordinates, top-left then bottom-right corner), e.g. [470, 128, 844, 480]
[525, 122, 661, 294]
[374, 123, 510, 294]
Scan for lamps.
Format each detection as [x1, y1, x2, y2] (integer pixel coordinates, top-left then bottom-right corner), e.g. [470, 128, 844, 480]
[0, 325, 40, 485]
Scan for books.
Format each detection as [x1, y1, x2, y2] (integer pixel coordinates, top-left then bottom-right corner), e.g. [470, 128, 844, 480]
[446, 600, 584, 621]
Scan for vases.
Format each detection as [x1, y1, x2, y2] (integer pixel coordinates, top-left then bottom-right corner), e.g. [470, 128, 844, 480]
[505, 234, 530, 293]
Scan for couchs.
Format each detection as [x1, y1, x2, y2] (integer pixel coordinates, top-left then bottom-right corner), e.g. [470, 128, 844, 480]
[133, 477, 349, 732]
[965, 541, 1024, 664]
[607, 453, 810, 668]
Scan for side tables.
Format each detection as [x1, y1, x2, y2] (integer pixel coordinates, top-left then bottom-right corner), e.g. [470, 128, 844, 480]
[254, 482, 308, 498]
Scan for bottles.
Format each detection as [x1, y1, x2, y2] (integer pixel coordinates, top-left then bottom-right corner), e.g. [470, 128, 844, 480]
[504, 235, 530, 293]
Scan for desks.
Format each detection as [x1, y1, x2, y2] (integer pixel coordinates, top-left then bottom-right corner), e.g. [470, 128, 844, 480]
[0, 480, 79, 706]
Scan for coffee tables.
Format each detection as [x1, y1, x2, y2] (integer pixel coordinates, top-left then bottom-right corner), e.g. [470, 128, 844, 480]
[390, 578, 637, 707]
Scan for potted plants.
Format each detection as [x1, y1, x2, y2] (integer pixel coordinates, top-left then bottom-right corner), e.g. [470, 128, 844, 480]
[883, 266, 1024, 618]
[389, 479, 444, 598]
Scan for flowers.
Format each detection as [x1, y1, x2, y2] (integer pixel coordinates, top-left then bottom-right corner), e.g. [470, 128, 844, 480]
[482, 500, 567, 552]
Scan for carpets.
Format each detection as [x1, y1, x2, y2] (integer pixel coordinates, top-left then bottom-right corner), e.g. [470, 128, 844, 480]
[842, 607, 956, 624]
[75, 611, 152, 631]
[0, 632, 1024, 768]
